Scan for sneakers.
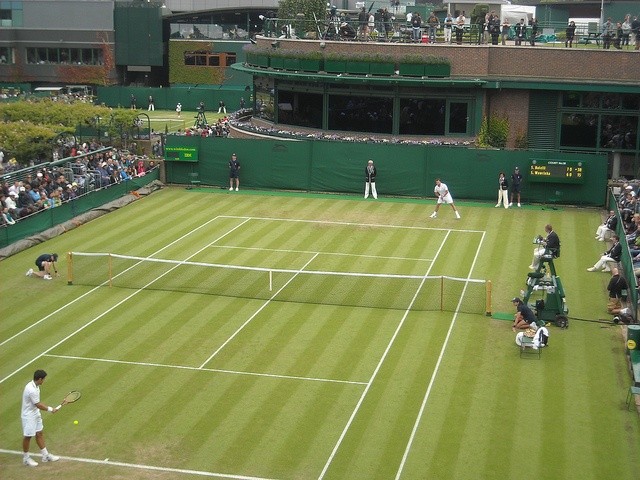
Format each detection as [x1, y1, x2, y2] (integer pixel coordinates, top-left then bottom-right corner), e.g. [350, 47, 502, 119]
[602, 268, 610, 272]
[599, 238, 604, 241]
[518, 203, 521, 207]
[495, 204, 501, 208]
[532, 322, 537, 331]
[587, 267, 596, 272]
[26, 268, 32, 276]
[595, 236, 600, 239]
[429, 214, 437, 218]
[505, 206, 508, 209]
[509, 203, 512, 206]
[41, 454, 59, 463]
[623, 308, 627, 316]
[229, 187, 233, 191]
[44, 275, 52, 280]
[23, 457, 38, 466]
[456, 214, 460, 219]
[236, 187, 238, 191]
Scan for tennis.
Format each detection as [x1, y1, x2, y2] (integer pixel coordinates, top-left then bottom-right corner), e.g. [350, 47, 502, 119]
[73, 420, 79, 426]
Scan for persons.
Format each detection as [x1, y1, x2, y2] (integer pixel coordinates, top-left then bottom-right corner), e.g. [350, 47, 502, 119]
[21, 370, 60, 466]
[176, 103, 182, 118]
[514, 19, 527, 45]
[148, 96, 155, 112]
[411, 12, 421, 43]
[341, 22, 357, 41]
[359, 7, 369, 40]
[491, 15, 501, 45]
[508, 167, 523, 207]
[488, 11, 496, 33]
[429, 179, 461, 219]
[217, 100, 227, 114]
[176, 116, 230, 138]
[495, 172, 508, 209]
[618, 184, 640, 276]
[603, 17, 614, 49]
[63, 140, 155, 187]
[595, 211, 617, 241]
[606, 268, 628, 315]
[0, 165, 79, 225]
[444, 14, 454, 44]
[228, 154, 240, 191]
[25, 253, 61, 280]
[630, 16, 640, 44]
[427, 11, 440, 44]
[154, 140, 161, 158]
[129, 94, 137, 110]
[530, 21, 537, 46]
[529, 224, 560, 270]
[482, 18, 489, 44]
[240, 97, 245, 109]
[510, 297, 537, 333]
[456, 13, 465, 45]
[368, 13, 374, 34]
[565, 21, 576, 48]
[614, 21, 623, 49]
[364, 160, 378, 199]
[502, 18, 511, 45]
[587, 235, 622, 272]
[622, 18, 631, 45]
[406, 12, 413, 22]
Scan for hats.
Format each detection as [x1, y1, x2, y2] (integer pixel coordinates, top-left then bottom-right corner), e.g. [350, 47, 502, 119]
[53, 253, 57, 262]
[37, 173, 43, 177]
[9, 191, 15, 195]
[510, 298, 520, 302]
[515, 166, 519, 170]
[625, 186, 632, 190]
[72, 182, 76, 186]
[20, 187, 25, 192]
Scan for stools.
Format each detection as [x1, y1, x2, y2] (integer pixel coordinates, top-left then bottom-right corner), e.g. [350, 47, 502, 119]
[626, 387, 640, 411]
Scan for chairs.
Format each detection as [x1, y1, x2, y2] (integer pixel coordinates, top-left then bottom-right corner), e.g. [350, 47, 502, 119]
[521, 329, 544, 360]
[606, 252, 622, 272]
[605, 219, 619, 240]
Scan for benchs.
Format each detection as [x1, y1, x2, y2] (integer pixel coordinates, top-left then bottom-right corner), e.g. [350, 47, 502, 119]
[507, 36, 544, 46]
[582, 37, 621, 49]
